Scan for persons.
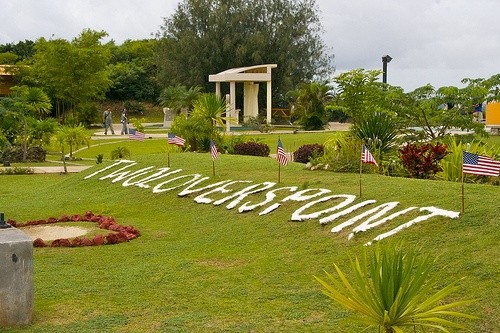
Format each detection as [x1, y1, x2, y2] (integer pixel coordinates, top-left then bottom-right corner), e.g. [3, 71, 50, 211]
[119, 106, 129, 135]
[103, 106, 115, 135]
[290, 112, 298, 132]
[441, 97, 487, 128]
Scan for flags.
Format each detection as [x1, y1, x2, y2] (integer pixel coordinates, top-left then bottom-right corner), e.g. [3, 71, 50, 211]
[463, 151, 500, 176]
[168, 131, 186, 145]
[129, 128, 145, 142]
[211, 138, 219, 161]
[361, 143, 379, 167]
[276, 139, 288, 166]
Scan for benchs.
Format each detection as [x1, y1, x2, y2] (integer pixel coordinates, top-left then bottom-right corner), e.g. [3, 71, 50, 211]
[486, 125, 500, 136]
